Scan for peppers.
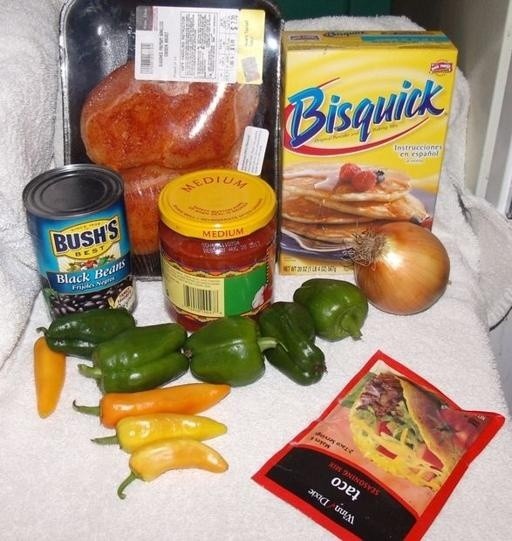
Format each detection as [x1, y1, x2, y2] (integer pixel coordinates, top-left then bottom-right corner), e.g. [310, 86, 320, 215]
[34, 277, 369, 499]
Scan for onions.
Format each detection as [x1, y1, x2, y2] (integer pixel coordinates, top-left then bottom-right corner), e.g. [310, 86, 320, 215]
[341, 221, 450, 316]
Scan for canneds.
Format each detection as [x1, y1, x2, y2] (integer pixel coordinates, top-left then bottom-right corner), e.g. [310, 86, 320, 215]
[157, 168, 279, 333]
[23, 163, 137, 319]
